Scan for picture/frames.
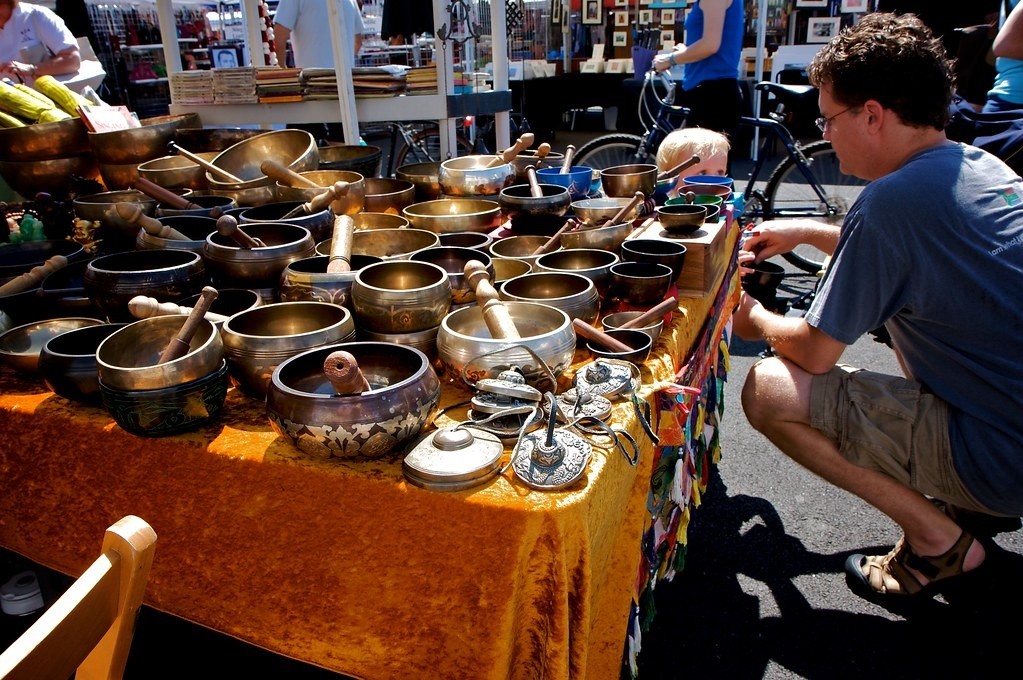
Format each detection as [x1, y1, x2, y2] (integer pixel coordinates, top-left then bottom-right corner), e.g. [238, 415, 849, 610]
[208, 44, 243, 69]
[552, 0, 869, 45]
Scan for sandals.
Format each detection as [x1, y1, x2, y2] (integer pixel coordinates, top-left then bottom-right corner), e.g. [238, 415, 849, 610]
[927, 497, 1022, 539]
[846, 528, 990, 610]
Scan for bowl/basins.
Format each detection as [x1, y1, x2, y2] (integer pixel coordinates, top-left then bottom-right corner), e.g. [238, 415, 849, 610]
[0, 112, 736, 461]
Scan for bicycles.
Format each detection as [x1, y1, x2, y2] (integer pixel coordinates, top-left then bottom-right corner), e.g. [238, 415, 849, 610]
[571, 46, 873, 277]
[314, 118, 476, 181]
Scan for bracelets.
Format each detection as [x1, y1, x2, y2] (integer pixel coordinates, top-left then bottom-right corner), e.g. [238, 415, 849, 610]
[669, 53, 677, 66]
[29, 63, 35, 77]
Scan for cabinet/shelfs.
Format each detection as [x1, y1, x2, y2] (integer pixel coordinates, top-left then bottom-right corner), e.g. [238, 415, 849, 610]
[127, 38, 212, 83]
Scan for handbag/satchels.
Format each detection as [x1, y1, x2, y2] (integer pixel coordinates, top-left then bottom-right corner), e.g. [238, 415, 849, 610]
[18, 6, 107, 95]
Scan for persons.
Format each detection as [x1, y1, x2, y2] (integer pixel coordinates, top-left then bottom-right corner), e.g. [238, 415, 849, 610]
[271, 0, 367, 149]
[653, 0, 746, 200]
[813, 22, 831, 36]
[655, 127, 755, 276]
[127, 60, 167, 82]
[970, 0, 1023, 164]
[0, 0, 81, 88]
[731, 12, 1023, 607]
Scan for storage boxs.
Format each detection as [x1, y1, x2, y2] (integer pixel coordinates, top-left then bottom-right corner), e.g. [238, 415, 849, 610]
[625, 219, 728, 294]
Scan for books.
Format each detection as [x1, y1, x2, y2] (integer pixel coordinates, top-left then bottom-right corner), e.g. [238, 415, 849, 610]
[168, 65, 491, 106]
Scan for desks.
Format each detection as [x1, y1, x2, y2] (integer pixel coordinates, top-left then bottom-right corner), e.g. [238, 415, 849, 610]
[1, 209, 747, 680]
[498, 70, 824, 140]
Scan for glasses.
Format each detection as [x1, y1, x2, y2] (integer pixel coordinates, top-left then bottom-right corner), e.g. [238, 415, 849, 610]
[814, 98, 884, 132]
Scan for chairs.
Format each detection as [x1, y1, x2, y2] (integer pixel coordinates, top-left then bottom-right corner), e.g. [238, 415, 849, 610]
[3, 515, 158, 679]
